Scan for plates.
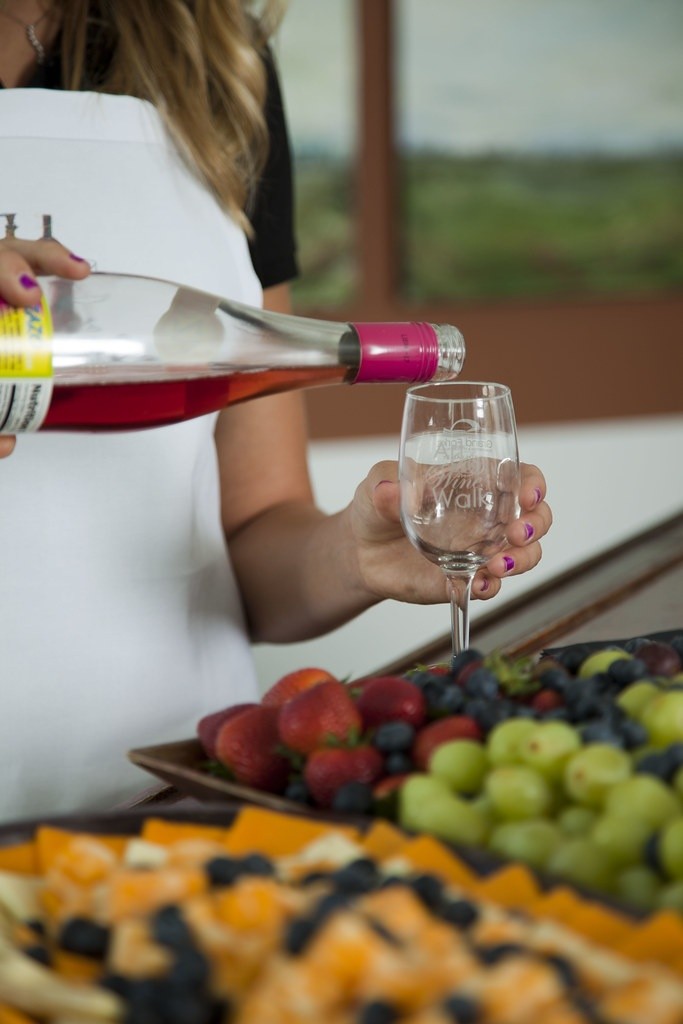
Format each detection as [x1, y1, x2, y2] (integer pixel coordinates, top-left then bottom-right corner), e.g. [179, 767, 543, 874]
[126, 735, 348, 820]
[0, 801, 240, 845]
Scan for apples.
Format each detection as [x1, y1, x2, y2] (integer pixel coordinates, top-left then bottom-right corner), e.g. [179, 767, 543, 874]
[400, 647, 683, 911]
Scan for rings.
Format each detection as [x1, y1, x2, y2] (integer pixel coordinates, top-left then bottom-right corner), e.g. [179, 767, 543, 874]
[496, 457, 511, 493]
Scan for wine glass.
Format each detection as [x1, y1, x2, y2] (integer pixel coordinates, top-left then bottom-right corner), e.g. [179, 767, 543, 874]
[395, 381, 521, 658]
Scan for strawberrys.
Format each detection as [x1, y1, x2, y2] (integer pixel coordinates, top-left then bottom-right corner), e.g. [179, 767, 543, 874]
[200, 666, 476, 801]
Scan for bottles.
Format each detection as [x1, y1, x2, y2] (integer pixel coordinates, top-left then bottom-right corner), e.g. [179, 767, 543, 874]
[0, 270, 467, 435]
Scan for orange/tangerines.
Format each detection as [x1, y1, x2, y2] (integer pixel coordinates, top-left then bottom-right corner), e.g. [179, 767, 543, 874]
[0, 806, 683, 1024]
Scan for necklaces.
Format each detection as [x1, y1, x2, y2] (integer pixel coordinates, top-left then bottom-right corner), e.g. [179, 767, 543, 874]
[1, 6, 52, 63]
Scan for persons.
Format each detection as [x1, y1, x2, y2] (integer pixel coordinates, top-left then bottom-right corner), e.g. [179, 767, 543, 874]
[0, 0, 554, 827]
